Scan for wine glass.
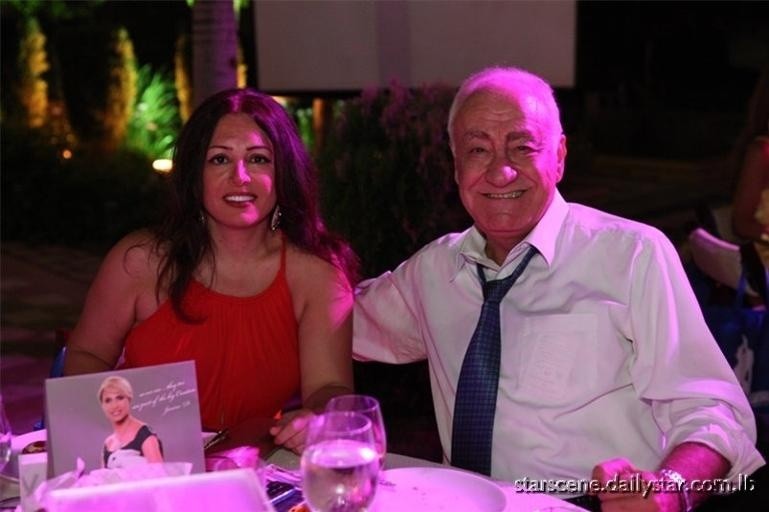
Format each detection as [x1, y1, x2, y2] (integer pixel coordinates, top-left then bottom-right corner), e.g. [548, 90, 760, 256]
[300, 395, 387, 512]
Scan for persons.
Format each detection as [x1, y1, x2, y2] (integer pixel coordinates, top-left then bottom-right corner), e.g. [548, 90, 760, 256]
[61, 87, 354, 457]
[350, 65, 766, 512]
[727, 73, 769, 309]
[97, 376, 164, 468]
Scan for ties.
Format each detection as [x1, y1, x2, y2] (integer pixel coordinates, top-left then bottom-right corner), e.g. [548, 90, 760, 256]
[452, 248, 537, 475]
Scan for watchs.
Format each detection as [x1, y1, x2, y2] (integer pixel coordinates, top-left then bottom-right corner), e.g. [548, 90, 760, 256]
[654, 468, 692, 512]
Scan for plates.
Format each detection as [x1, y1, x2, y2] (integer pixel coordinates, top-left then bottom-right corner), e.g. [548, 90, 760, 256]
[367, 466, 508, 512]
